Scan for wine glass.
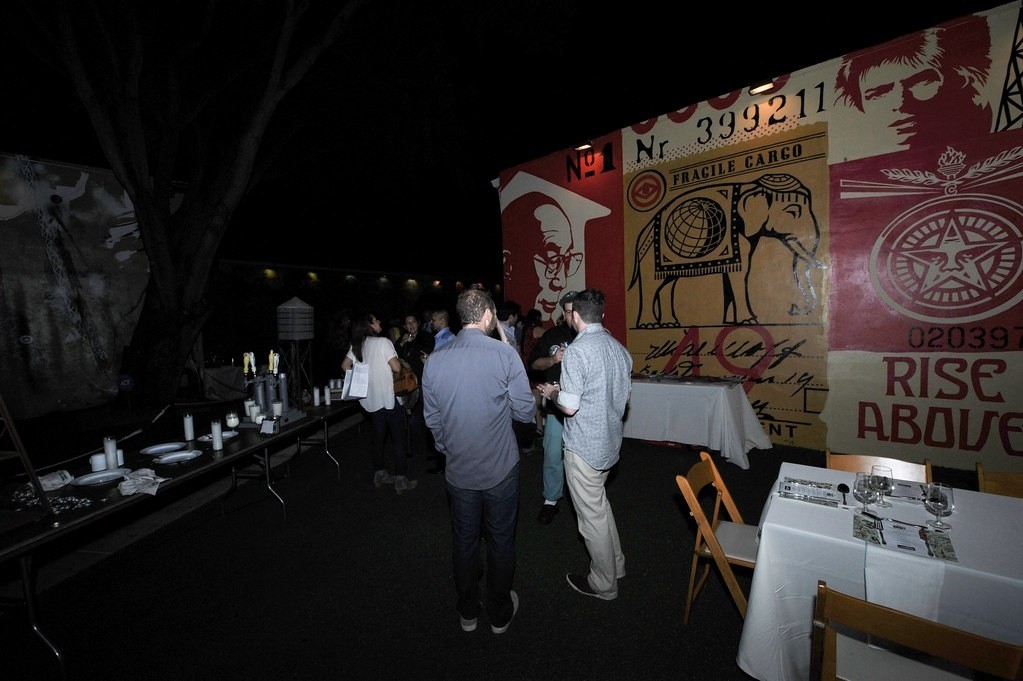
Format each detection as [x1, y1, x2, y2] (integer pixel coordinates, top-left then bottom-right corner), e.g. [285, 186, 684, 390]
[925, 482, 954, 529]
[853, 472, 878, 517]
[225, 412, 239, 431]
[870, 466, 893, 507]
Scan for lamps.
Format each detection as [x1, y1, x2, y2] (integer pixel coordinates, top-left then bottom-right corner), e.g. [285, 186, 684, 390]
[749, 78, 775, 95]
[574, 139, 594, 152]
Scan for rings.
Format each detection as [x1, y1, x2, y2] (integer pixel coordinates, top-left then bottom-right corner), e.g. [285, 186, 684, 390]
[541, 388, 544, 391]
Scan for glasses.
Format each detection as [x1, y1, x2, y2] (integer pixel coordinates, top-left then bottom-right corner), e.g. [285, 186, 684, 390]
[565, 310, 572, 314]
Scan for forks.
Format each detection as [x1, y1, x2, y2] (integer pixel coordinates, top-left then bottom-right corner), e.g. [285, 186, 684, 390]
[874, 519, 886, 545]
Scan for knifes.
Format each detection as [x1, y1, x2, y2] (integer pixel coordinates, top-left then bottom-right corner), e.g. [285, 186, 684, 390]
[919, 529, 934, 557]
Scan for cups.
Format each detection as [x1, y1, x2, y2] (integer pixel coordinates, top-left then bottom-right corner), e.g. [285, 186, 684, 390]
[210, 419, 224, 451]
[244, 373, 289, 425]
[102, 434, 119, 469]
[406, 332, 413, 342]
[549, 381, 560, 387]
[324, 384, 332, 406]
[313, 386, 320, 406]
[183, 412, 195, 442]
[560, 341, 570, 348]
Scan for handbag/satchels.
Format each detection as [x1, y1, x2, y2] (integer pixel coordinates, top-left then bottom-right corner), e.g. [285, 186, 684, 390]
[395, 358, 419, 395]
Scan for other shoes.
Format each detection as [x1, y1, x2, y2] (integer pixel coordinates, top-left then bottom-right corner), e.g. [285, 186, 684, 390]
[374, 469, 395, 488]
[395, 475, 417, 495]
[522, 442, 535, 454]
[423, 464, 445, 474]
[537, 502, 560, 525]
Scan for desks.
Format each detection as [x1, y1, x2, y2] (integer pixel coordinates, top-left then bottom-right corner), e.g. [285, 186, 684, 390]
[736, 460, 1023, 681]
[0, 390, 367, 681]
[620, 376, 772, 471]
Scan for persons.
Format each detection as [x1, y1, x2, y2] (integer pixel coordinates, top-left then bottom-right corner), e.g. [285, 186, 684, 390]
[421, 310, 456, 365]
[529, 292, 580, 521]
[497, 301, 548, 456]
[395, 313, 435, 381]
[422, 289, 536, 633]
[535, 289, 627, 601]
[341, 313, 418, 493]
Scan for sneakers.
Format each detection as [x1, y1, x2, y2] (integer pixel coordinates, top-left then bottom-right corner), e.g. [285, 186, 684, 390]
[492, 591, 519, 633]
[460, 616, 478, 631]
[566, 573, 610, 601]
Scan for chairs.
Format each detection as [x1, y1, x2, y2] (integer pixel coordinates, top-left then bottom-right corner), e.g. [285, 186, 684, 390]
[675, 451, 759, 627]
[826, 448, 933, 484]
[810, 580, 1023, 681]
[975, 461, 1023, 499]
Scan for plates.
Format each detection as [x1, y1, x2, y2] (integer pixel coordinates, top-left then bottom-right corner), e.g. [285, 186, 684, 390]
[69, 469, 132, 486]
[140, 442, 203, 465]
[196, 431, 240, 443]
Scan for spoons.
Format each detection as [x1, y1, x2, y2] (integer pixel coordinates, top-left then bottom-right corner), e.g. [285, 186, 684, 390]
[837, 483, 850, 505]
[862, 511, 929, 529]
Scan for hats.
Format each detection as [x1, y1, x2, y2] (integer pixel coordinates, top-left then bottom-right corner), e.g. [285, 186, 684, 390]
[559, 291, 580, 306]
